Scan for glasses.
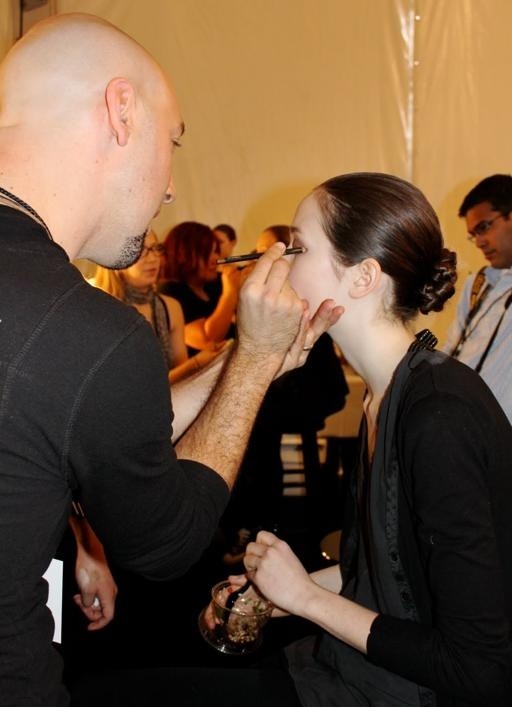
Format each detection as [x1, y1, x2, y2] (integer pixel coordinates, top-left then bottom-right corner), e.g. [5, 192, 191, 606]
[466, 214, 502, 243]
[141, 244, 167, 258]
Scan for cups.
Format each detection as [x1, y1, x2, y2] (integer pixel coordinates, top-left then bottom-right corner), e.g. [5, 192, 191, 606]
[200, 580, 273, 658]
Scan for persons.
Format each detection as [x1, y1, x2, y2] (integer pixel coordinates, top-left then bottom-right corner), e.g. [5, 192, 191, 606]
[2, 8, 346, 704]
[44, 220, 350, 707]
[439, 173, 512, 432]
[200, 169, 512, 706]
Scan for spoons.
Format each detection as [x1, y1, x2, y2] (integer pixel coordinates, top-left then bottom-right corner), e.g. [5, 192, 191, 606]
[221, 572, 257, 624]
